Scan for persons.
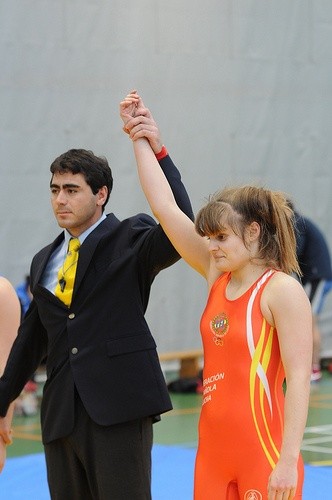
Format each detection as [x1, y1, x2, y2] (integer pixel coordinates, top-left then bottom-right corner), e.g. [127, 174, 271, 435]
[0, 272, 48, 475]
[0, 96, 195, 499]
[271, 198, 332, 383]
[120, 88, 312, 500]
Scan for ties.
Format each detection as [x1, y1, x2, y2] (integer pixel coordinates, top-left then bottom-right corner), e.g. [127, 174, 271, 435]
[55, 238, 80, 308]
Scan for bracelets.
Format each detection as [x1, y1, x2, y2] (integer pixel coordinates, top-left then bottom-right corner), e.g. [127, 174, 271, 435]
[155, 145, 168, 160]
